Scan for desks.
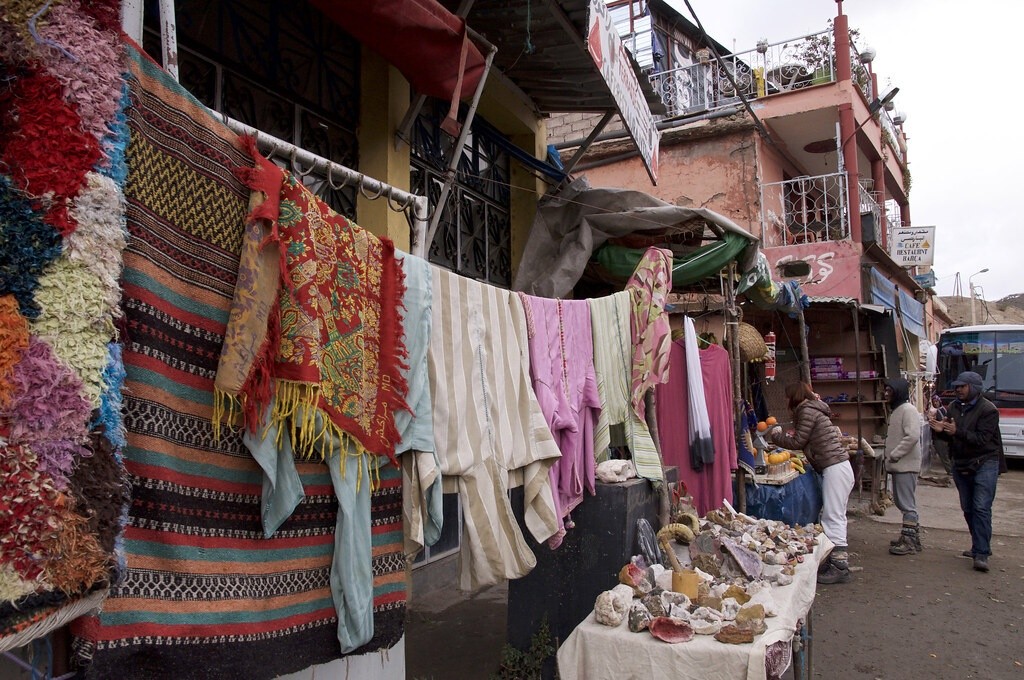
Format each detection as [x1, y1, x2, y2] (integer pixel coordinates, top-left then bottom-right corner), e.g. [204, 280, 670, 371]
[842, 449, 884, 503]
[554, 530, 838, 680]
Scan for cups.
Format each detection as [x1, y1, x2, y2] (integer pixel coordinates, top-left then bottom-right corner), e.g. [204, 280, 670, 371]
[672, 569, 699, 600]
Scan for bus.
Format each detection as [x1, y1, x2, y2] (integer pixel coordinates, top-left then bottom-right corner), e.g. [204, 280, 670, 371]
[933, 325, 1024, 458]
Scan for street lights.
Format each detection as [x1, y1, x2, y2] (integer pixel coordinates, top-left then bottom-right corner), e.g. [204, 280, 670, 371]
[969, 268, 989, 325]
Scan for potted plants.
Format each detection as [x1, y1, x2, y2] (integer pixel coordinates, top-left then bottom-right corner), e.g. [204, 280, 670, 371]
[885, 102, 894, 112]
[695, 48, 709, 65]
[860, 52, 875, 64]
[755, 38, 767, 53]
[782, 18, 862, 86]
[893, 117, 903, 125]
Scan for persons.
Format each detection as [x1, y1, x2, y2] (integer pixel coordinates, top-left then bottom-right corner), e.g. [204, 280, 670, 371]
[929, 370, 1001, 572]
[773, 381, 856, 584]
[931, 394, 953, 475]
[883, 377, 924, 555]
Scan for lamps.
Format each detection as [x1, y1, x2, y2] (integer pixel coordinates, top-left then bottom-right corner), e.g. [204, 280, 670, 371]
[840, 88, 900, 152]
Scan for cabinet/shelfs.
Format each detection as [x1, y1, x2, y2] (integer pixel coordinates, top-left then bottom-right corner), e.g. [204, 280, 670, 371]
[506, 465, 681, 674]
[811, 345, 889, 492]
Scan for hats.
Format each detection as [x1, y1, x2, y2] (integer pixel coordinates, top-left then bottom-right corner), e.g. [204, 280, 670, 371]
[950, 372, 983, 402]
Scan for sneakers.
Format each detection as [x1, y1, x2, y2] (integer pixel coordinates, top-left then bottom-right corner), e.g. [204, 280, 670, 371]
[818, 557, 851, 584]
[963, 545, 992, 571]
[890, 532, 922, 554]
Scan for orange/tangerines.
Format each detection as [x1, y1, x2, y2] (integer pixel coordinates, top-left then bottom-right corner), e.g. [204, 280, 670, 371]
[752, 448, 790, 464]
[756, 417, 777, 432]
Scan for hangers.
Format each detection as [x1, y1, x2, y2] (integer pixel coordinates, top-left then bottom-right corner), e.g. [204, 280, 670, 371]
[684, 318, 712, 346]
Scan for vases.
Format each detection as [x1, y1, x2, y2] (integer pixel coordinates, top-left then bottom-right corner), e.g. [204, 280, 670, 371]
[765, 64, 808, 95]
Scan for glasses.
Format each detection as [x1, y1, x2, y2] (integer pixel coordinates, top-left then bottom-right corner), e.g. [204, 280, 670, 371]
[885, 388, 891, 392]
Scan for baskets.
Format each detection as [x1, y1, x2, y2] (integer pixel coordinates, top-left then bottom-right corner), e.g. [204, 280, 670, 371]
[735, 306, 774, 363]
